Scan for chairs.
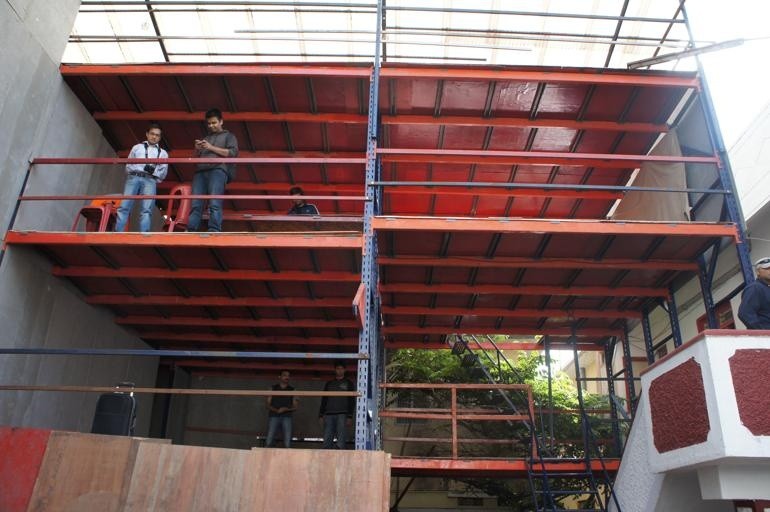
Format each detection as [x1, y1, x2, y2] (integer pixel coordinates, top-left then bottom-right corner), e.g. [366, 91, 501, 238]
[159, 184, 193, 232]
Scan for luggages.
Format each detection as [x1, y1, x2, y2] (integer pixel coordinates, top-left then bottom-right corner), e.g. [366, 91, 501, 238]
[92, 382, 136, 436]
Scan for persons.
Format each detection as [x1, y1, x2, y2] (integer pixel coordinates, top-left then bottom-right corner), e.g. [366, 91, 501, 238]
[115, 124, 170, 232]
[185, 109, 237, 232]
[319, 363, 355, 449]
[288, 188, 320, 215]
[264, 369, 299, 448]
[738, 257, 770, 330]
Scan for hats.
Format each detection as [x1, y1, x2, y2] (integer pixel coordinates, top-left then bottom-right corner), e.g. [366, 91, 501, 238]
[754, 256, 770, 269]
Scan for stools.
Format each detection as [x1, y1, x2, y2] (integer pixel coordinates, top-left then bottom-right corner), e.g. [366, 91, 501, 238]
[71, 203, 117, 232]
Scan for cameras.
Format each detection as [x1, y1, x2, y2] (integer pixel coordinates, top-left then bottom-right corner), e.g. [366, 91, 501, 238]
[144, 165, 155, 173]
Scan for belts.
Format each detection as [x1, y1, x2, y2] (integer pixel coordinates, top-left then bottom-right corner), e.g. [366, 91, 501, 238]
[128, 171, 153, 177]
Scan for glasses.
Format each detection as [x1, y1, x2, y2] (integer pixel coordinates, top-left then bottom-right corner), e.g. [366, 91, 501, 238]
[758, 259, 770, 264]
